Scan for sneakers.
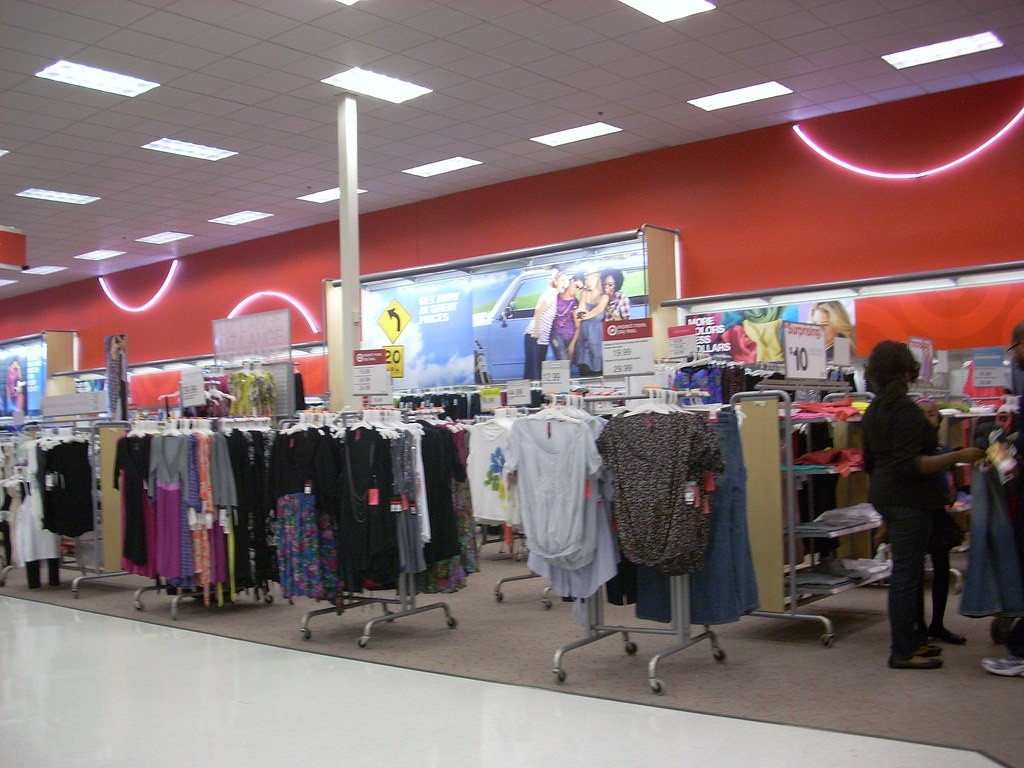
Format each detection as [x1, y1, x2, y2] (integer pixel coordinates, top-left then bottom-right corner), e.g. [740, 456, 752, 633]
[981, 654, 1024, 677]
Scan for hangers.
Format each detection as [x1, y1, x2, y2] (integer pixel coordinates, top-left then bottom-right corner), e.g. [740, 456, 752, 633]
[0, 352, 782, 454]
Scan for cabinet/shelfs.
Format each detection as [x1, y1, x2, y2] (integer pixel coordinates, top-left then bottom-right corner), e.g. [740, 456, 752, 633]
[780, 414, 893, 595]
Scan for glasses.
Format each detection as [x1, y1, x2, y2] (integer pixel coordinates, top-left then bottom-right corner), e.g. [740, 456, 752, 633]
[573, 281, 581, 290]
[603, 282, 616, 286]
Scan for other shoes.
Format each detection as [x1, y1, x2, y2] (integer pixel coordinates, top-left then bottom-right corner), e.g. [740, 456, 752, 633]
[927, 625, 967, 645]
[912, 645, 942, 657]
[888, 655, 944, 670]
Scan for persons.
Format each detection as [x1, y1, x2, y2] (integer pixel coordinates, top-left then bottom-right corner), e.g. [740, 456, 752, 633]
[810, 301, 856, 360]
[982, 320, 1024, 676]
[524, 268, 631, 405]
[861, 339, 987, 668]
[6, 355, 22, 412]
